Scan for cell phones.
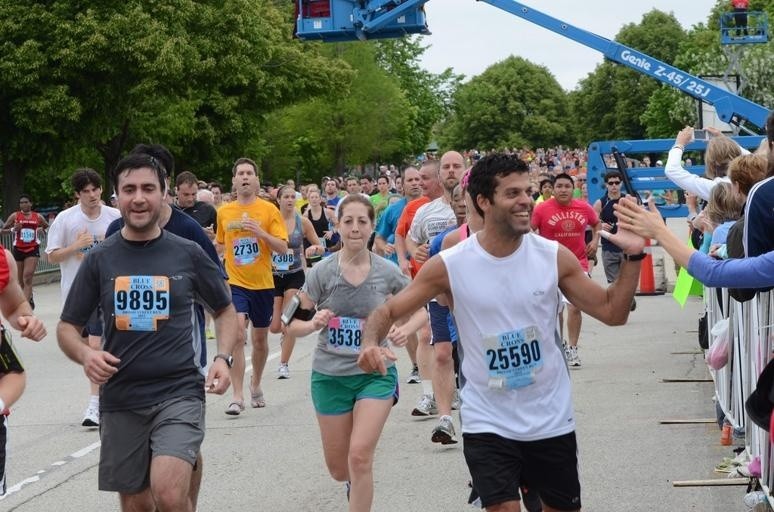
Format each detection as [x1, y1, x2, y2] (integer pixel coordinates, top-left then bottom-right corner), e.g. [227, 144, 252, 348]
[692, 130, 709, 141]
[207, 227, 213, 231]
[280, 295, 299, 326]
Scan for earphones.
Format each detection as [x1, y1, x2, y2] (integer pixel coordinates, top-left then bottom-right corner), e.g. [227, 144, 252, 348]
[174, 187, 177, 194]
[337, 227, 341, 236]
[114, 191, 119, 200]
[371, 229, 373, 237]
[161, 194, 166, 200]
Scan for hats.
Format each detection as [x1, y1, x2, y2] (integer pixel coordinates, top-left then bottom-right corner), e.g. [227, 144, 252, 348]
[321, 176, 332, 182]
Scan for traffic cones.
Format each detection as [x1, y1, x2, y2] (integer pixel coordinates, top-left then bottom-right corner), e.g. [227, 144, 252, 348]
[635, 235, 664, 298]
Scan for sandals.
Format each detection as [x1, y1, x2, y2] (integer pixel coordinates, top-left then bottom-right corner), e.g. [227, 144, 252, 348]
[250, 384, 265, 410]
[226, 400, 246, 418]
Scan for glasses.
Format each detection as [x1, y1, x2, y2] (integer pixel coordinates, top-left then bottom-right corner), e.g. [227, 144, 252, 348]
[606, 181, 622, 186]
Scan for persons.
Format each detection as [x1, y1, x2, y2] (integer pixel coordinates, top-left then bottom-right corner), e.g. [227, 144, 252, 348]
[730, 0, 749, 35]
[0, 113, 774, 512]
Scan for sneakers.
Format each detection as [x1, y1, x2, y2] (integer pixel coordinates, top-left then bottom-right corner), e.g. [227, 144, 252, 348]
[450, 395, 463, 410]
[205, 329, 217, 341]
[277, 364, 291, 380]
[411, 394, 438, 417]
[432, 414, 458, 445]
[407, 368, 422, 384]
[81, 407, 102, 427]
[564, 344, 584, 368]
